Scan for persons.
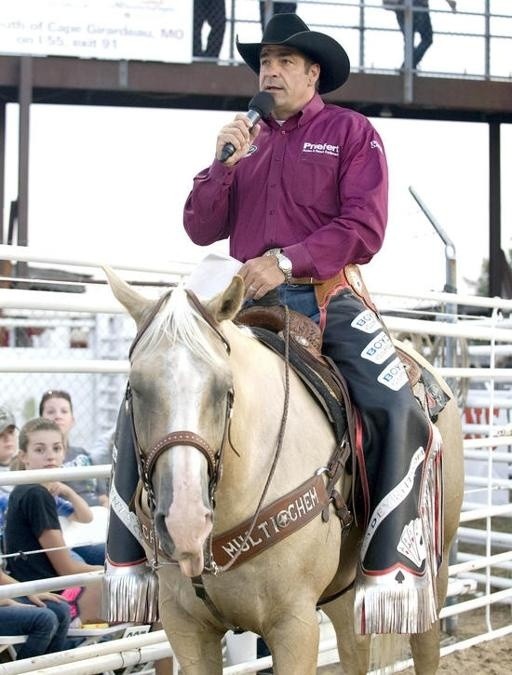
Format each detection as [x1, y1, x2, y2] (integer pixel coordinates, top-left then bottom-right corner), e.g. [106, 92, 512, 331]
[1, 573, 72, 660]
[192, 0, 226, 65]
[1, 390, 110, 622]
[381, 0, 457, 76]
[260, 1, 297, 34]
[96, 15, 445, 638]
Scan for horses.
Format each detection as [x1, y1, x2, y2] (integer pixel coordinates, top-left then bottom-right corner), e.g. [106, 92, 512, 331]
[98, 263, 466, 675]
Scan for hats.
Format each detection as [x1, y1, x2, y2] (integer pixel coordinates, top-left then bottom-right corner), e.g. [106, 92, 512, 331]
[229, 10, 355, 99]
[0, 403, 21, 437]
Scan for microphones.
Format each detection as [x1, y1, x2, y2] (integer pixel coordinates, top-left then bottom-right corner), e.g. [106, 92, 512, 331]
[219, 90, 276, 163]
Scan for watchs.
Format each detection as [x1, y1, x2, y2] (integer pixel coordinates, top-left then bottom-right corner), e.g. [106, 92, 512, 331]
[275, 253, 293, 282]
